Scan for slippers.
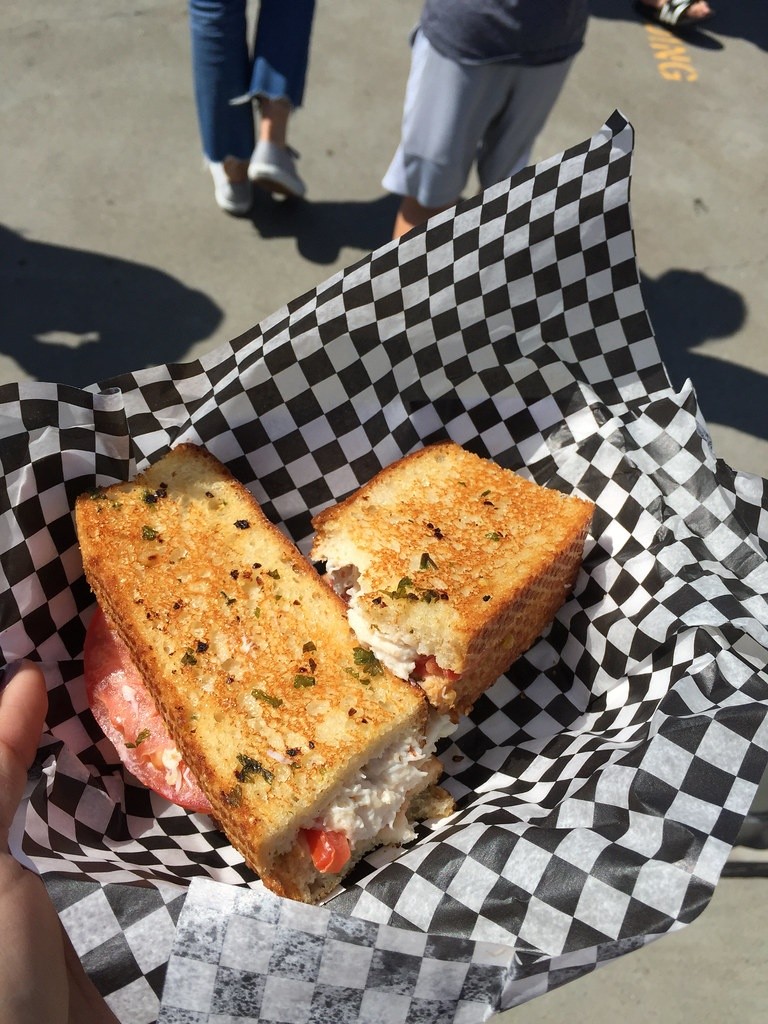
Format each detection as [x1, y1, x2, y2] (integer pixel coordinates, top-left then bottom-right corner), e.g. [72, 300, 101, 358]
[632, 0, 717, 30]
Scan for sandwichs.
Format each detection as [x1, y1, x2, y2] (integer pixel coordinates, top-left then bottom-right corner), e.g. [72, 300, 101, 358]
[310, 442, 594, 714]
[69, 443, 451, 906]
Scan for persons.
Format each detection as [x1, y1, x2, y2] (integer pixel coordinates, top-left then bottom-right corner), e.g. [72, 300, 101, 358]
[382, 1, 593, 240]
[187, 0, 315, 214]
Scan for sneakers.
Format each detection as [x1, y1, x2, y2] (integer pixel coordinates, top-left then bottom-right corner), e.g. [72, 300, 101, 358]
[209, 158, 250, 212]
[248, 143, 306, 199]
